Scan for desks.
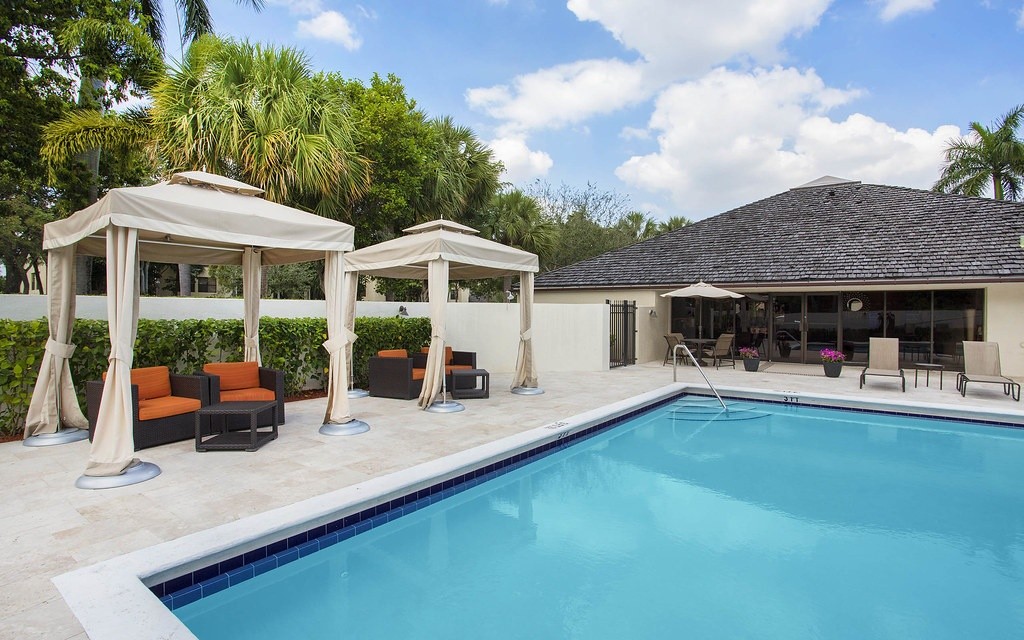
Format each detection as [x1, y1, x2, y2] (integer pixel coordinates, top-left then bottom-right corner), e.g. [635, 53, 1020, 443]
[914, 364, 944, 390]
[450, 369, 490, 400]
[195, 400, 281, 452]
[681, 339, 716, 366]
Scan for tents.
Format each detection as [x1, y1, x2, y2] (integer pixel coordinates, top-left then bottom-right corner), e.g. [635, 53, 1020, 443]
[343, 219, 546, 413]
[23, 169, 369, 489]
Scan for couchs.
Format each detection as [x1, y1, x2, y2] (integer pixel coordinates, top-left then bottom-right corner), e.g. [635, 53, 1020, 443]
[421, 347, 477, 391]
[369, 349, 429, 400]
[86, 366, 209, 452]
[192, 362, 286, 438]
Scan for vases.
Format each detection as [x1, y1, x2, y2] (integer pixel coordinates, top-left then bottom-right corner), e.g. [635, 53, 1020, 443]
[823, 359, 844, 377]
[744, 357, 760, 371]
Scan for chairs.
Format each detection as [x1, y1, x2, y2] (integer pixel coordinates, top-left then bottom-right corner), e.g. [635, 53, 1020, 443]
[956, 341, 1021, 401]
[705, 333, 736, 369]
[663, 333, 696, 366]
[861, 337, 905, 392]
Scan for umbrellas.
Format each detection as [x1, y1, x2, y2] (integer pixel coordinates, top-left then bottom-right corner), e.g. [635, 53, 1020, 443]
[660, 279, 745, 363]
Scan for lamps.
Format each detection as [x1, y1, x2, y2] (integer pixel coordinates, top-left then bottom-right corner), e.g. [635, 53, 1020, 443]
[399, 306, 408, 315]
[648, 310, 657, 319]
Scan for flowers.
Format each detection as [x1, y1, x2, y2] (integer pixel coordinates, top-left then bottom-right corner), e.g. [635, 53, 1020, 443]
[739, 347, 761, 359]
[818, 348, 845, 362]
